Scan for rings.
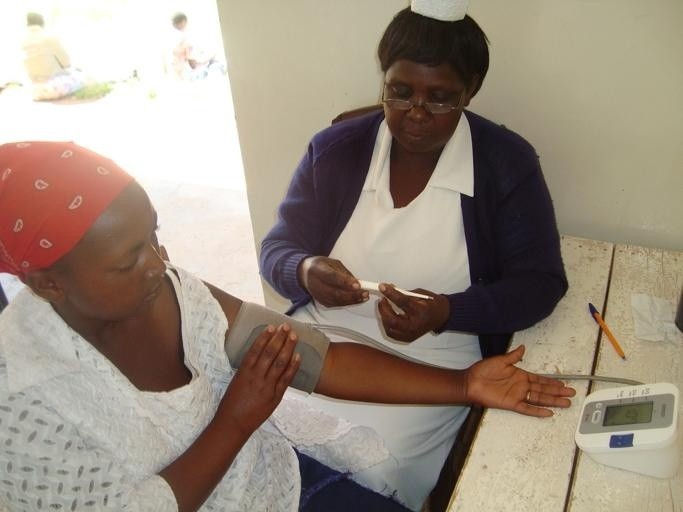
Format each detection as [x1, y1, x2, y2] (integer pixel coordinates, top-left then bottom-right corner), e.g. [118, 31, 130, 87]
[525, 389, 532, 402]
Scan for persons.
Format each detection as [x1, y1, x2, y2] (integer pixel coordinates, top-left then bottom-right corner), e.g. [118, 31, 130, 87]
[242, 0, 569, 512]
[16, 11, 85, 102]
[164, 13, 216, 78]
[0, 137, 578, 512]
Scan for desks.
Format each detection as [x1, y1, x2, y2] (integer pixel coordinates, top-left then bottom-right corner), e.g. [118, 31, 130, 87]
[444, 235, 683, 512]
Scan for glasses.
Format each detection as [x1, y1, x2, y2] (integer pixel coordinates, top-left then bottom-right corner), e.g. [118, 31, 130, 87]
[380, 82, 468, 115]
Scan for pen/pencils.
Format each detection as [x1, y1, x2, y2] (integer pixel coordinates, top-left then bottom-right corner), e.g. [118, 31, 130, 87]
[587, 302, 626, 363]
